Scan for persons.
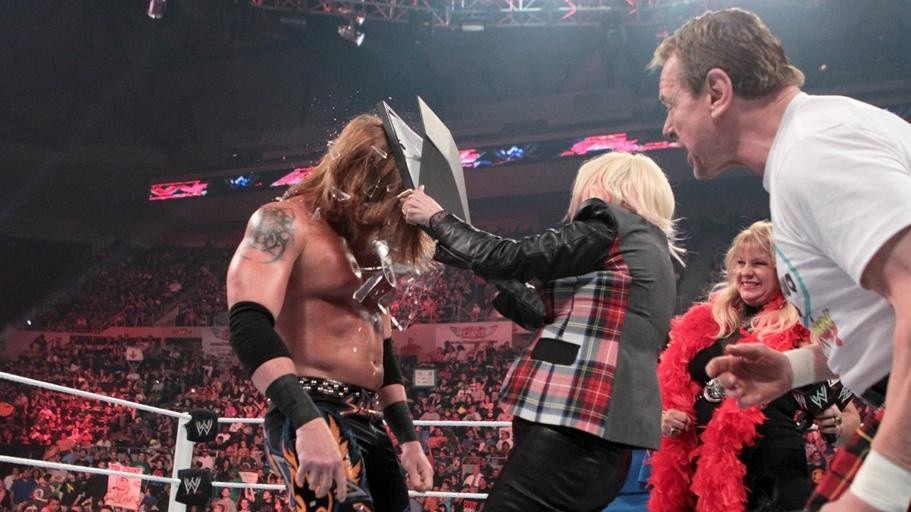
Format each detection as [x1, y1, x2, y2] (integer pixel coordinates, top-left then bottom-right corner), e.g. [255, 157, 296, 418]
[659, 218, 862, 511]
[651, 4, 911, 512]
[225, 107, 437, 511]
[397, 149, 682, 511]
[794, 398, 879, 484]
[0, 223, 726, 512]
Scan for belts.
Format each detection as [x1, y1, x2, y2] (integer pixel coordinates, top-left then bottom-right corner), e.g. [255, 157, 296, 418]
[266, 373, 380, 412]
